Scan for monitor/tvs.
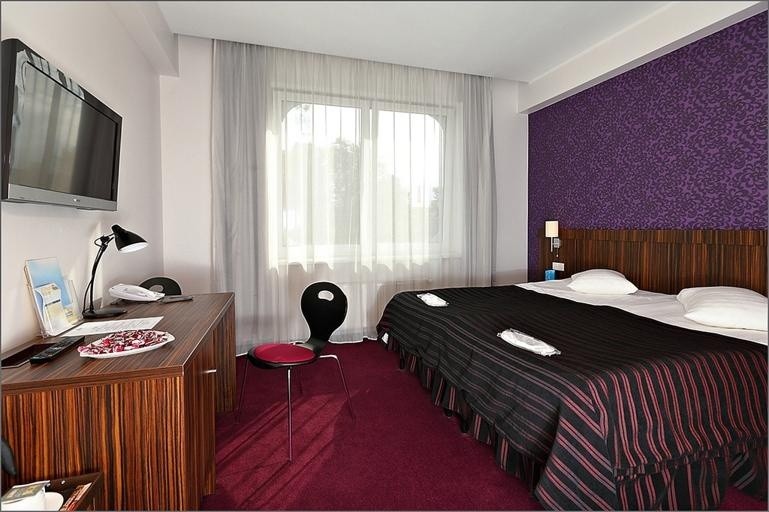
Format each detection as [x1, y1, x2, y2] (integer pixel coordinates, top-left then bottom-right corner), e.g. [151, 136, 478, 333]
[0, 38, 121, 210]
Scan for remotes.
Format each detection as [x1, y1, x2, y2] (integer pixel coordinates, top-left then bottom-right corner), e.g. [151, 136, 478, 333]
[30, 335, 84, 365]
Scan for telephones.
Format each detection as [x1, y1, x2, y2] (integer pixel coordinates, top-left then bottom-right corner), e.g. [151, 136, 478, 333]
[108, 282, 164, 301]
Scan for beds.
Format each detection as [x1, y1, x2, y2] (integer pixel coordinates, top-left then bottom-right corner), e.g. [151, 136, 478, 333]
[374, 228, 769, 510]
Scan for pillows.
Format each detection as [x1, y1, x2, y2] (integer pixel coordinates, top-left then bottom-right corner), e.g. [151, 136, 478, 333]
[675, 286, 768, 331]
[565, 269, 639, 295]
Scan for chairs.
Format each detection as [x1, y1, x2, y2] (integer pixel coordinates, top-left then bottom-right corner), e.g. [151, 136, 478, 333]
[236, 282, 356, 464]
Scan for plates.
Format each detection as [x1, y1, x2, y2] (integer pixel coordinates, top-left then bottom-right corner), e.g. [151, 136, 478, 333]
[79, 327, 175, 361]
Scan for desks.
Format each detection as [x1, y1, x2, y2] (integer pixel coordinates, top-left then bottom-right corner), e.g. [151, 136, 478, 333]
[0, 291, 236, 510]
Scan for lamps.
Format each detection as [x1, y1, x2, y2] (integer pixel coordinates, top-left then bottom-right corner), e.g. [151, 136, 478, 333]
[81, 224, 150, 319]
[545, 220, 564, 258]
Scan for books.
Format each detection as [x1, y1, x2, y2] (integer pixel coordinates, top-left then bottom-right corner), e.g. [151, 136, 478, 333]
[1, 479, 52, 511]
[25, 257, 72, 334]
